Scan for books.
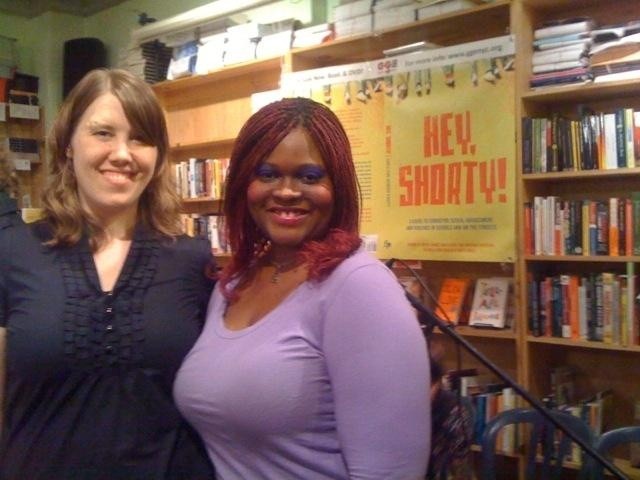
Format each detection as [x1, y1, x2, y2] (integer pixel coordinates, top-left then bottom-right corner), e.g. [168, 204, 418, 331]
[1, 74, 43, 223]
[167, 159, 236, 253]
[379, 260, 636, 461]
[521, 16, 639, 257]
[120, 0, 476, 82]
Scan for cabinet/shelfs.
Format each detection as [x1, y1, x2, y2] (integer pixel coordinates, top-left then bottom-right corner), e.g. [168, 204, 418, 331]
[151, 1, 640, 479]
[0, 100, 48, 210]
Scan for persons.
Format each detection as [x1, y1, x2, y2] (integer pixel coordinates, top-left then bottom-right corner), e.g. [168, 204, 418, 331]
[430, 357, 472, 480]
[173, 99, 430, 480]
[0, 68, 215, 480]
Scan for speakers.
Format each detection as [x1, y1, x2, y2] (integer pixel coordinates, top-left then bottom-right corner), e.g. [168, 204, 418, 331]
[62, 38, 105, 102]
